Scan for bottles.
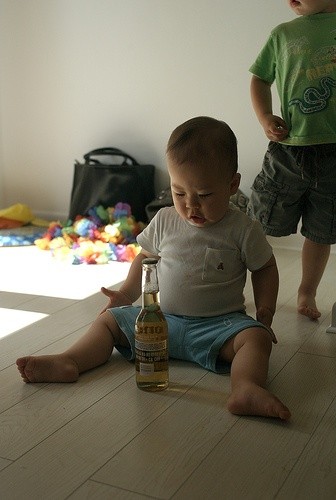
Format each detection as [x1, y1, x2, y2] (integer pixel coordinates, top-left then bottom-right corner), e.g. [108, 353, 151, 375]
[135, 256, 169, 392]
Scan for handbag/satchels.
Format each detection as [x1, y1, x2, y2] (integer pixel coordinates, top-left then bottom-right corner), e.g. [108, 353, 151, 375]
[69, 148, 155, 225]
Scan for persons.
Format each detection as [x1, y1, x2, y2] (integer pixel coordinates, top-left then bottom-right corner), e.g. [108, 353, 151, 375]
[16, 116, 291, 419]
[245, 0, 336, 320]
